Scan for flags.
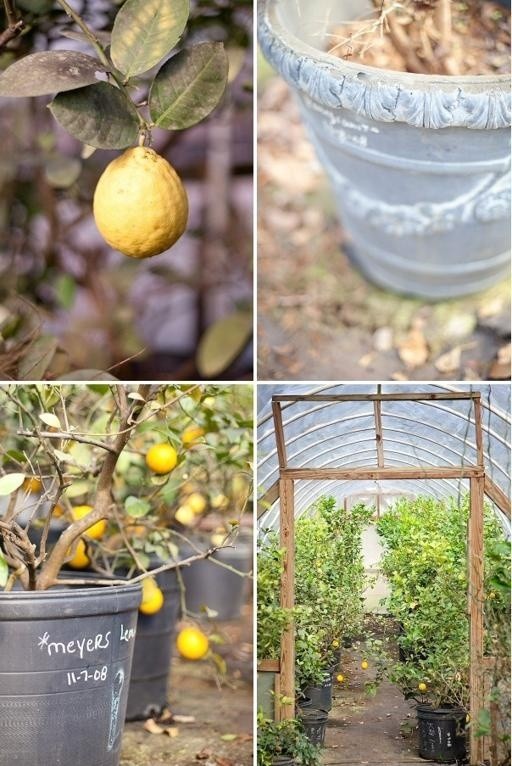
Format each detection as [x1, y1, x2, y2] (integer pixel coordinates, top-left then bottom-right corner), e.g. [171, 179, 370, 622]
[57, 559, 182, 720]
[395, 619, 467, 762]
[18, 518, 70, 558]
[0, 569, 142, 765]
[176, 540, 249, 621]
[259, 636, 353, 766]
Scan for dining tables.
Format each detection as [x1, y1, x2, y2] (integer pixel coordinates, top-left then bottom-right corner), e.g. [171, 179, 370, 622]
[256, 0, 512, 303]
